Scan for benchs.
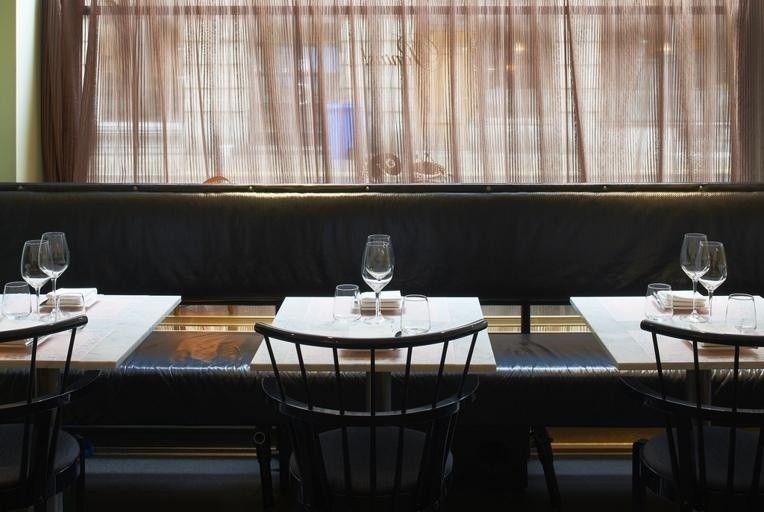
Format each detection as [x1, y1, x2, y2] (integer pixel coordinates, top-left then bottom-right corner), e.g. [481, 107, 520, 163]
[2, 180, 764, 426]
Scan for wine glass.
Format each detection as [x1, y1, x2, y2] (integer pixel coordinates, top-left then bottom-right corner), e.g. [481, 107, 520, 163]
[362, 233, 394, 332]
[21, 231, 70, 326]
[680, 232, 728, 331]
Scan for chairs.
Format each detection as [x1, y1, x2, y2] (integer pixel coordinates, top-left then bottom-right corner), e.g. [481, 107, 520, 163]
[0, 311, 103, 512]
[242, 316, 495, 510]
[614, 314, 764, 511]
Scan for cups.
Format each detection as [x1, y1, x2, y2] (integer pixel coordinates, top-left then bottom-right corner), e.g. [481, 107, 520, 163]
[55, 295, 88, 331]
[3, 282, 30, 324]
[333, 284, 361, 325]
[724, 294, 758, 335]
[644, 282, 674, 326]
[401, 294, 430, 333]
